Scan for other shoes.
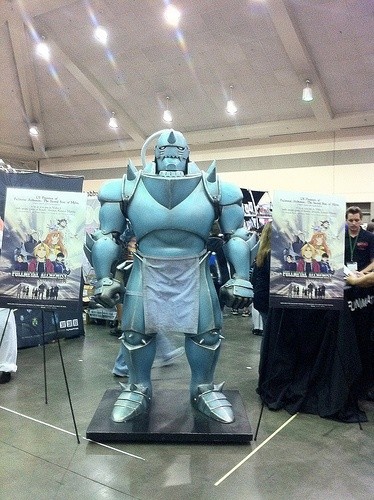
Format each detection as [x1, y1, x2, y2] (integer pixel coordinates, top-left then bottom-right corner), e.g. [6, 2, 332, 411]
[252, 329, 263, 335]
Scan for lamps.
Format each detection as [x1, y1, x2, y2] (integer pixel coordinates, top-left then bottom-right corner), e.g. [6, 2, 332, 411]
[302, 80, 314, 102]
[28, 117, 40, 138]
[225, 85, 239, 114]
[164, 96, 173, 122]
[109, 111, 119, 128]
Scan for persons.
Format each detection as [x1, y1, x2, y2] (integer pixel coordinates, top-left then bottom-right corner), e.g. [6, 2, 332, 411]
[113, 233, 186, 378]
[109, 237, 137, 339]
[210, 202, 374, 338]
